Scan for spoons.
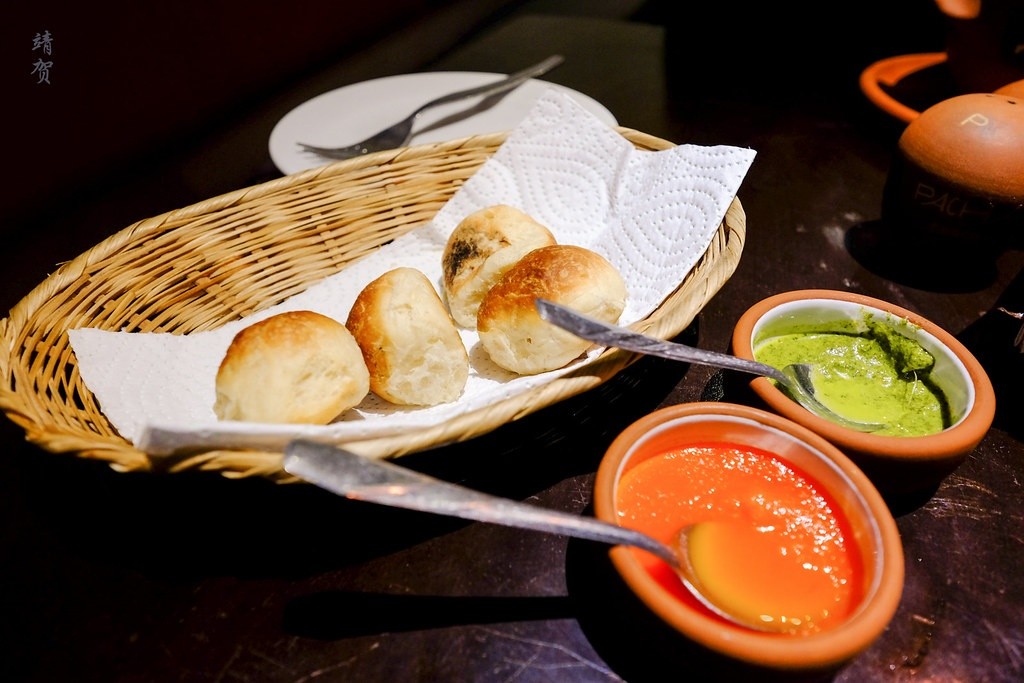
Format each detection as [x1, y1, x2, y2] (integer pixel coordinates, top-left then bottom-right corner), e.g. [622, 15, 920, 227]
[534, 293, 889, 434]
[282, 437, 844, 634]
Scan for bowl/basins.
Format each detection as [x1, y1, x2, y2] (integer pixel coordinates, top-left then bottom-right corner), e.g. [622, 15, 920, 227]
[594, 397, 907, 664]
[734, 287, 997, 457]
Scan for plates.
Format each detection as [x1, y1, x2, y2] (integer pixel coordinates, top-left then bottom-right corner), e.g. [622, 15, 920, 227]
[266, 71, 619, 176]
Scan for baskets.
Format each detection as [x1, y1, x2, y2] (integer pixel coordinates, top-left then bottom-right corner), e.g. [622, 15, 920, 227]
[0, 122, 748, 484]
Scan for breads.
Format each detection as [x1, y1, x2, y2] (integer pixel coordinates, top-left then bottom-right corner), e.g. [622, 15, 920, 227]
[476, 243, 627, 374]
[346, 269, 469, 405]
[214, 313, 371, 424]
[442, 205, 558, 325]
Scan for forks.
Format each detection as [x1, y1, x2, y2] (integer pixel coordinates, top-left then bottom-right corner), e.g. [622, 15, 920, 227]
[297, 54, 565, 170]
